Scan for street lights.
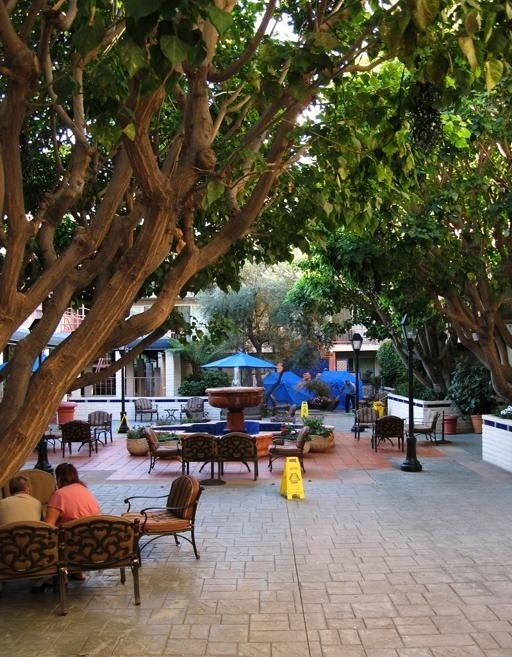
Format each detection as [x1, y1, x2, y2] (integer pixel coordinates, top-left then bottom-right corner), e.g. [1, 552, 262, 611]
[28, 317, 54, 478]
[348, 331, 367, 432]
[117, 342, 129, 433]
[398, 312, 425, 473]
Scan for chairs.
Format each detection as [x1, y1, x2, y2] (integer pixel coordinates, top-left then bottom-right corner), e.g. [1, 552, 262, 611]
[270, 391, 290, 417]
[216, 432, 260, 481]
[180, 435, 218, 479]
[42, 430, 61, 453]
[144, 425, 181, 473]
[135, 398, 159, 422]
[88, 411, 113, 444]
[179, 397, 206, 422]
[355, 407, 376, 441]
[407, 410, 440, 446]
[0, 521, 66, 615]
[268, 425, 310, 472]
[371, 415, 406, 454]
[122, 474, 204, 564]
[60, 420, 98, 457]
[60, 513, 142, 616]
[4, 469, 57, 517]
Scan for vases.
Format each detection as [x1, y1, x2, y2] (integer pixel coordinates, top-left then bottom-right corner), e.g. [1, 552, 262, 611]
[439, 416, 459, 434]
[58, 402, 77, 427]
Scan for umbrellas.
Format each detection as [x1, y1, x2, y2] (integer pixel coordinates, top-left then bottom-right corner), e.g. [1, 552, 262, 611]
[317, 369, 363, 411]
[200, 349, 278, 386]
[255, 371, 315, 412]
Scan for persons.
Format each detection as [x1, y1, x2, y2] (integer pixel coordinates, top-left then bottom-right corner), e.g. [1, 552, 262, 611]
[340, 379, 356, 414]
[44, 462, 101, 586]
[0, 475, 49, 593]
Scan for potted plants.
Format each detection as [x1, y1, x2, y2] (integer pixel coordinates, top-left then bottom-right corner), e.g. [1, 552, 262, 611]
[302, 415, 332, 453]
[127, 425, 149, 456]
[448, 360, 502, 435]
